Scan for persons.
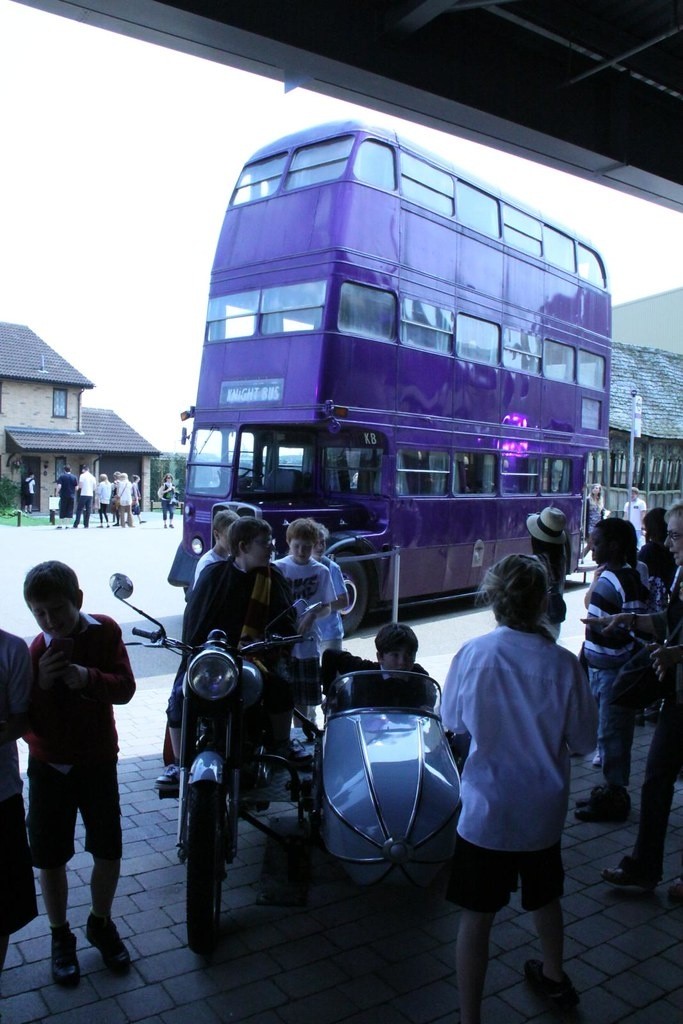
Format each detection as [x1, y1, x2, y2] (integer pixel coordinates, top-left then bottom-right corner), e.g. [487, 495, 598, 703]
[55, 465, 147, 529]
[154, 474, 683, 898]
[435, 551, 582, 1024]
[25, 473, 35, 513]
[15, 562, 131, 988]
[0, 630, 40, 969]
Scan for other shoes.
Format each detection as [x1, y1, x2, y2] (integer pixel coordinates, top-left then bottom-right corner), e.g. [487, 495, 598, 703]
[602, 856, 663, 889]
[164, 524, 175, 528]
[113, 524, 119, 526]
[592, 750, 601, 766]
[86, 913, 131, 971]
[576, 783, 631, 824]
[634, 708, 660, 726]
[525, 959, 580, 1011]
[51, 923, 80, 989]
[667, 878, 683, 898]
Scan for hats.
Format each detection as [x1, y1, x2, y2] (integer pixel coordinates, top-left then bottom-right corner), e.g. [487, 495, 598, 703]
[526, 506, 568, 545]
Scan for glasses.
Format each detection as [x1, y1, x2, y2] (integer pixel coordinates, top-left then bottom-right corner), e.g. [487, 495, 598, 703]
[667, 531, 679, 540]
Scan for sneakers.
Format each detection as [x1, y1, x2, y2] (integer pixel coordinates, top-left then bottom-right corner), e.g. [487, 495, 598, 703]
[155, 763, 181, 789]
[276, 739, 312, 762]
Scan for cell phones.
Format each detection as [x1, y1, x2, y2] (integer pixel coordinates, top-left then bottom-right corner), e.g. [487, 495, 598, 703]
[49, 638, 74, 664]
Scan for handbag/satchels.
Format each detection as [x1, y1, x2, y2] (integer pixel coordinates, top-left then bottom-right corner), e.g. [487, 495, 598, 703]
[114, 501, 120, 508]
[548, 593, 566, 623]
[607, 645, 667, 709]
[49, 496, 61, 510]
[132, 505, 140, 515]
[94, 498, 101, 510]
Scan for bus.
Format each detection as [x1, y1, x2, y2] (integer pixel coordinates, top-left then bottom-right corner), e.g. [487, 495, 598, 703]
[167, 120, 614, 637]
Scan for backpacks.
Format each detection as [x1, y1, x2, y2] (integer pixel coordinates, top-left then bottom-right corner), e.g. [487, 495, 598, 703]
[23, 479, 33, 495]
[158, 487, 163, 499]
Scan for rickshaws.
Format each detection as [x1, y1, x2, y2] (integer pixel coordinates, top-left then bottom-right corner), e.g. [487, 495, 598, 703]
[110, 574, 471, 953]
